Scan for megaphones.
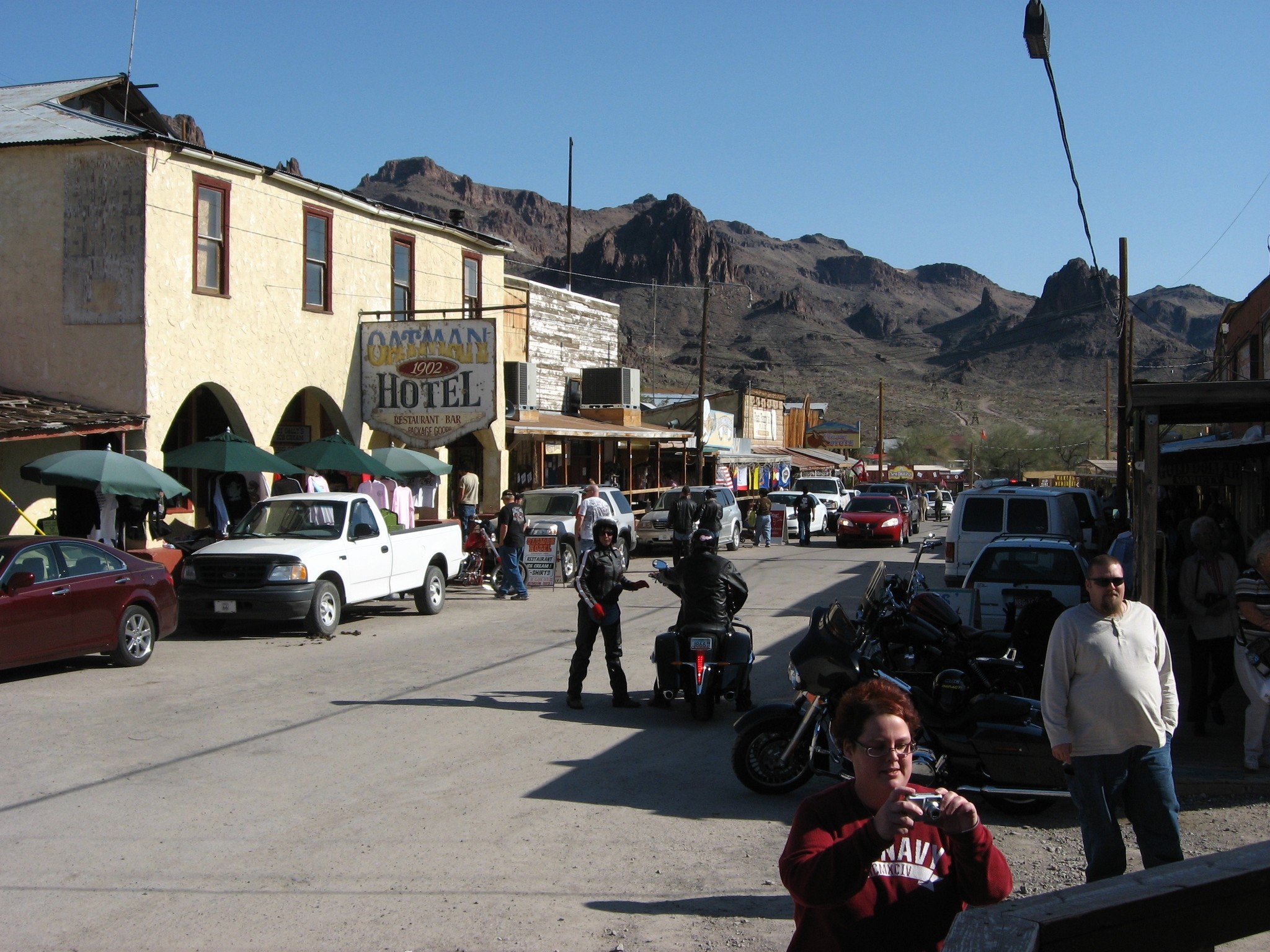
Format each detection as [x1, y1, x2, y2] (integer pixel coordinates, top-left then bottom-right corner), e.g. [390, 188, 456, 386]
[505, 400, 516, 418]
[668, 419, 680, 429]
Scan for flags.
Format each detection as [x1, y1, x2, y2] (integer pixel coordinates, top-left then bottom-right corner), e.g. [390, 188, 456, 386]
[982, 430, 986, 439]
[715, 463, 791, 492]
[850, 458, 868, 482]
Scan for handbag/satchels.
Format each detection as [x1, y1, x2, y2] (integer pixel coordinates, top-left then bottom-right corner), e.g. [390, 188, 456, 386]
[1242, 659, 1270, 705]
[1245, 638, 1270, 677]
[748, 510, 756, 527]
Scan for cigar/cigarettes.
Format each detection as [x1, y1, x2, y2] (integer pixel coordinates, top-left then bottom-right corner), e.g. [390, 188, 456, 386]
[1062, 761, 1067, 765]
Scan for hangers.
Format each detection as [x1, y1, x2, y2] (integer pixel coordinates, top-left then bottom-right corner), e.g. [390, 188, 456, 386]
[312, 470, 318, 477]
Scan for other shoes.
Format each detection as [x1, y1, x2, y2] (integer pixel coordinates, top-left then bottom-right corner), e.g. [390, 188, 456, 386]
[765, 544, 770, 547]
[753, 542, 758, 546]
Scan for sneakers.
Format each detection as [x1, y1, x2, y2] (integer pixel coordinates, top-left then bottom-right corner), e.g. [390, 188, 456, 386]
[494, 593, 506, 598]
[511, 593, 529, 600]
[1244, 755, 1259, 770]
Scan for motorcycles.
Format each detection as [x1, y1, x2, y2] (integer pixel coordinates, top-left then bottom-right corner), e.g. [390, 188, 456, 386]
[650, 560, 754, 724]
[731, 533, 1075, 825]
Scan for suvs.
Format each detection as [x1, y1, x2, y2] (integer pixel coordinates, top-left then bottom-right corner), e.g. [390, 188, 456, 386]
[636, 484, 743, 556]
[852, 482, 922, 537]
[515, 484, 638, 584]
[961, 531, 1089, 633]
[789, 475, 851, 528]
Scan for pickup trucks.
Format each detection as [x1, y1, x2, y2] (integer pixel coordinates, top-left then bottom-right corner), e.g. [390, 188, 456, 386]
[176, 492, 470, 636]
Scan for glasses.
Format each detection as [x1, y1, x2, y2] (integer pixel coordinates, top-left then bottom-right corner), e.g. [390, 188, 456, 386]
[1089, 577, 1125, 588]
[599, 530, 616, 536]
[849, 738, 917, 758]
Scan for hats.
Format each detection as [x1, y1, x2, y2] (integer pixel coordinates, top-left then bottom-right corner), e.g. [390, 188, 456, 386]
[514, 492, 524, 502]
[500, 490, 514, 499]
[579, 488, 588, 494]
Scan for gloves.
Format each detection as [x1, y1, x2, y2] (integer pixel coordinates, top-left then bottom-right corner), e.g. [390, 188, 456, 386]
[633, 580, 649, 589]
[592, 604, 604, 621]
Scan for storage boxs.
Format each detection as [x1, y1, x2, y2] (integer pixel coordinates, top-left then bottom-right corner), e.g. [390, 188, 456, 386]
[652, 633, 680, 689]
[720, 632, 752, 690]
[972, 716, 1065, 785]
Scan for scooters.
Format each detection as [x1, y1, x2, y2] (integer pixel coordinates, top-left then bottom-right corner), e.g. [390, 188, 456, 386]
[444, 513, 530, 596]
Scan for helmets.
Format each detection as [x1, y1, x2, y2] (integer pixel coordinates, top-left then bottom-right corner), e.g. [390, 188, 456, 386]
[592, 515, 618, 544]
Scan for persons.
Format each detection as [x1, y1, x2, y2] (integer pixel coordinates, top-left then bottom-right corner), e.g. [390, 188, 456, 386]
[1097, 484, 1248, 619]
[566, 519, 649, 710]
[456, 464, 480, 548]
[494, 489, 528, 600]
[631, 465, 678, 510]
[750, 488, 772, 547]
[664, 486, 723, 567]
[779, 679, 1013, 952]
[793, 485, 815, 546]
[647, 528, 756, 712]
[1180, 516, 1241, 738]
[917, 485, 929, 520]
[930, 485, 943, 522]
[1040, 553, 1184, 884]
[566, 484, 614, 588]
[1234, 529, 1270, 770]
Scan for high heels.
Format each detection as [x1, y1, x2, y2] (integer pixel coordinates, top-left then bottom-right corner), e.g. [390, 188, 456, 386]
[567, 695, 583, 710]
[611, 694, 641, 708]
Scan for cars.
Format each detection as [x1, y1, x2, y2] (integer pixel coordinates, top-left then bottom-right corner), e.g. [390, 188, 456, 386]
[924, 490, 955, 521]
[836, 492, 911, 548]
[767, 491, 828, 539]
[846, 489, 862, 499]
[0, 535, 182, 671]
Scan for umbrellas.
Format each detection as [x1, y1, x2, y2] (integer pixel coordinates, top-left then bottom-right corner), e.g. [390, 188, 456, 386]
[19, 425, 453, 552]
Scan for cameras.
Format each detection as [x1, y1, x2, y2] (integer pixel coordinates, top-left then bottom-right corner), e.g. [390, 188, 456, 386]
[905, 792, 944, 821]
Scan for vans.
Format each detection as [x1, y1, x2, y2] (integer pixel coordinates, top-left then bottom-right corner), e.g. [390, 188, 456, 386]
[944, 477, 1121, 589]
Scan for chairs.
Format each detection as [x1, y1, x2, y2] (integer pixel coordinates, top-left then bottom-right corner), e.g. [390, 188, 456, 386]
[69, 555, 104, 576]
[996, 558, 1025, 579]
[22, 556, 48, 583]
